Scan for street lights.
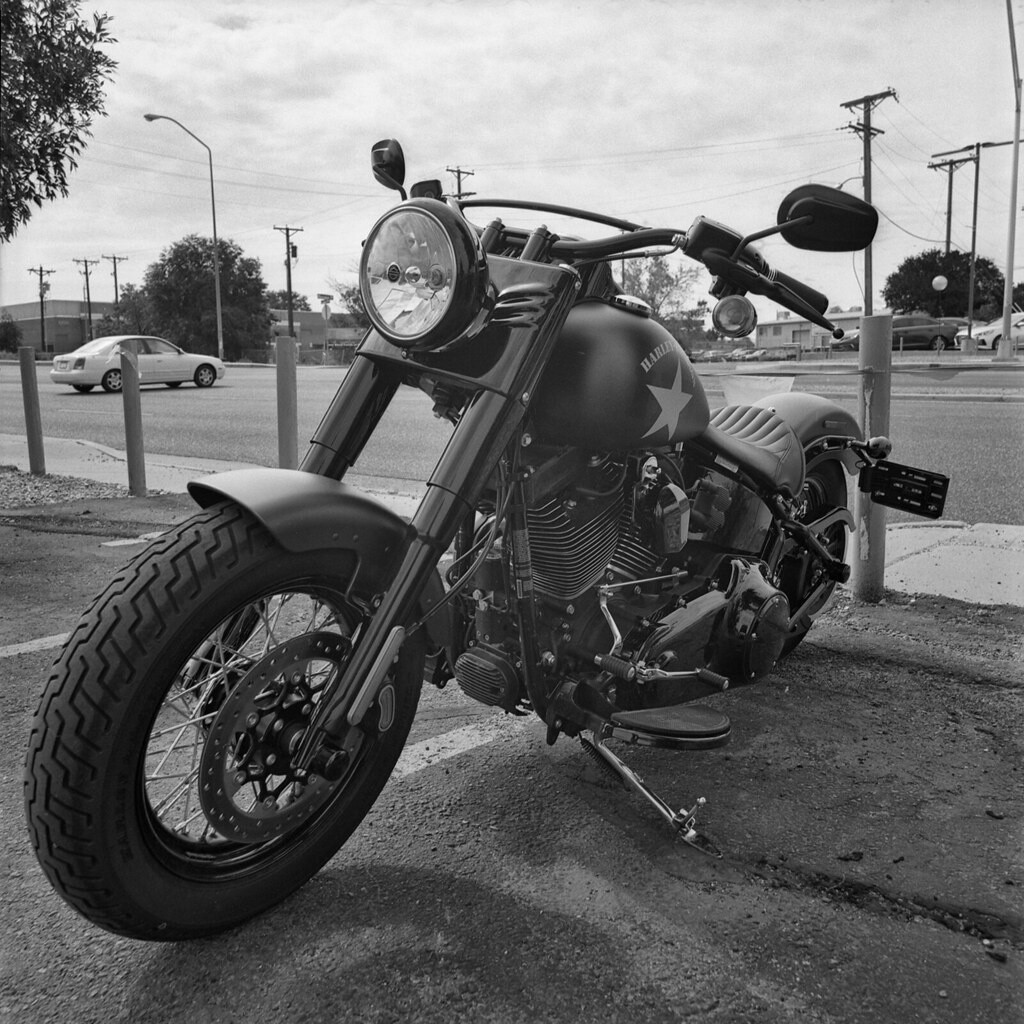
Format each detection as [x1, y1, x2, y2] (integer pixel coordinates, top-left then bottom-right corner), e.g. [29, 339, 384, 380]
[143, 111, 226, 363]
[931, 275, 949, 316]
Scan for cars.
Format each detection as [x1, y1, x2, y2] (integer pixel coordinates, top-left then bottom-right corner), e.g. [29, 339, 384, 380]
[953, 311, 1024, 351]
[687, 348, 796, 362]
[50, 334, 226, 393]
[934, 318, 977, 334]
[827, 314, 959, 352]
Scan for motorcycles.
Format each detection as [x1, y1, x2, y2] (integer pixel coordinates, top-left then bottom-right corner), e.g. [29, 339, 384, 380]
[22, 137, 953, 946]
